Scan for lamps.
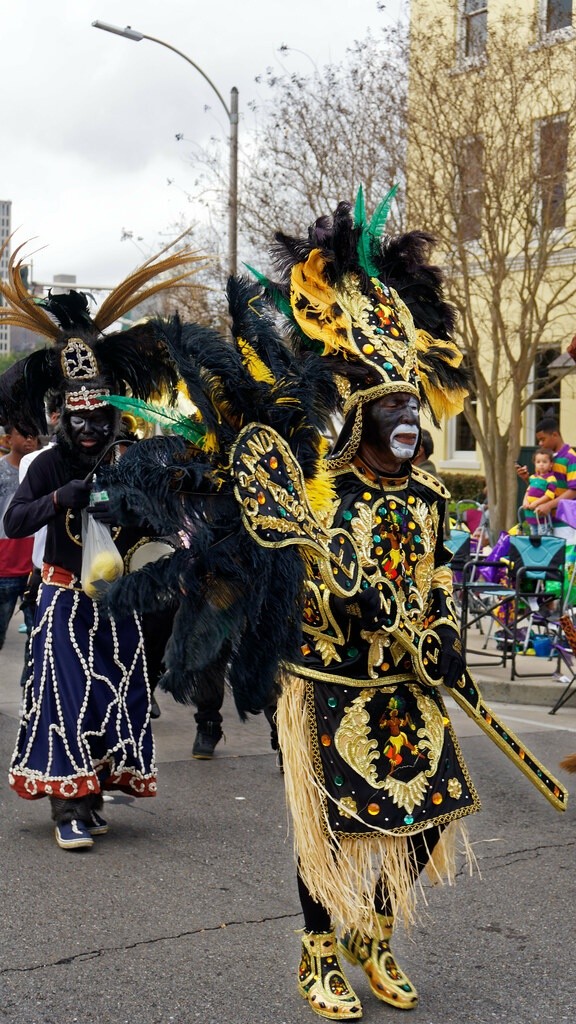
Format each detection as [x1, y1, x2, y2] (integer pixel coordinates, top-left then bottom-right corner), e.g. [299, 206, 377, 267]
[547, 334, 576, 378]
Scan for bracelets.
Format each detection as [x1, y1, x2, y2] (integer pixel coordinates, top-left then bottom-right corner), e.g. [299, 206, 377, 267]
[54, 490, 57, 503]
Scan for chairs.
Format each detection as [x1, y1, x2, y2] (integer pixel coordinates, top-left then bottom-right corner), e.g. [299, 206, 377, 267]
[460, 534, 576, 715]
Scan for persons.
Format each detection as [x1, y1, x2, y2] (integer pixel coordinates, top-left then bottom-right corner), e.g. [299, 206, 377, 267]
[522, 449, 557, 535]
[0, 355, 575, 1024]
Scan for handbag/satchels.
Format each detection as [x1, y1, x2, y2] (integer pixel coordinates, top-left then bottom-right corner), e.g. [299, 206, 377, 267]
[81, 484, 123, 601]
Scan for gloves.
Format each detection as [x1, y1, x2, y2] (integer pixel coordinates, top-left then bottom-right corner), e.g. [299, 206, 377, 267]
[57, 478, 89, 512]
[86, 497, 118, 526]
[331, 587, 387, 632]
[431, 624, 467, 687]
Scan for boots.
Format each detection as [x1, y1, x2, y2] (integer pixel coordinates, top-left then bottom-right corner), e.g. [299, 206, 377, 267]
[298, 929, 365, 1019]
[337, 893, 420, 1009]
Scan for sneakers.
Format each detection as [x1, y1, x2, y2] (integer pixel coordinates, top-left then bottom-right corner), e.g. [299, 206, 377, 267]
[55, 819, 93, 849]
[75, 808, 108, 835]
[192, 726, 226, 758]
